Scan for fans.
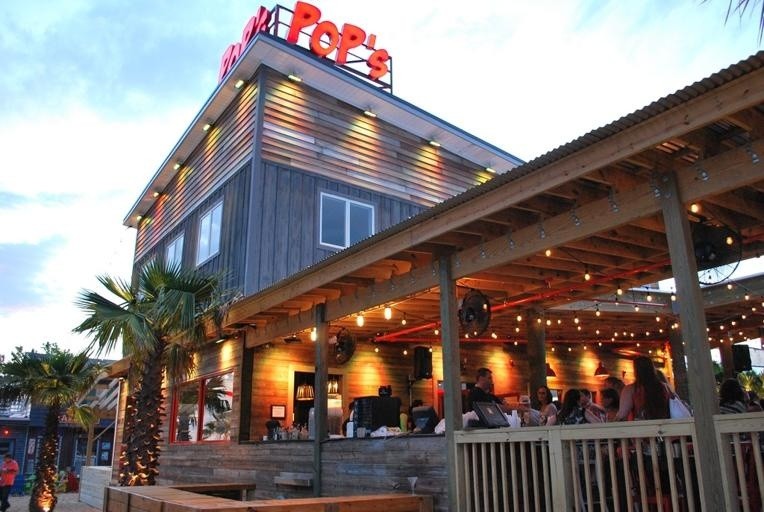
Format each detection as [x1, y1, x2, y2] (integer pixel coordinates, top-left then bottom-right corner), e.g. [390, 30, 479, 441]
[460, 290, 490, 339]
[690, 219, 742, 286]
[332, 327, 356, 365]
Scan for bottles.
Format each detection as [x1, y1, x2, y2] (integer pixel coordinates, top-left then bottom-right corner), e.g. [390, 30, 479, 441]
[512, 410, 517, 428]
[400, 410, 408, 432]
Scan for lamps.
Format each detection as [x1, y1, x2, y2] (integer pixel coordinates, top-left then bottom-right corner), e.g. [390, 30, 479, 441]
[545, 348, 612, 378]
[296, 373, 314, 401]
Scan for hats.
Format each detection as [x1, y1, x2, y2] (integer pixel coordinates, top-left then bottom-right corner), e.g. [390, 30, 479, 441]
[519, 395, 530, 404]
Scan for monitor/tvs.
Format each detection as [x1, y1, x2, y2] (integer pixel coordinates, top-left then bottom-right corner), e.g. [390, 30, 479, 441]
[473, 401, 511, 428]
[411, 405, 439, 434]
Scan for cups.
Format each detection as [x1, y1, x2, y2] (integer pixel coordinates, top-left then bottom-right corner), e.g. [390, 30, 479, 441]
[357, 428, 366, 438]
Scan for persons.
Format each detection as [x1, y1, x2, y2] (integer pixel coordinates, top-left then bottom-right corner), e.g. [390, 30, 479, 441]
[0, 453, 19, 512]
[466, 357, 763, 494]
[342, 397, 426, 436]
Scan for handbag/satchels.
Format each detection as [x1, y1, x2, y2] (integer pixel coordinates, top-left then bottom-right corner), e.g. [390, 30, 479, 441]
[669, 396, 692, 418]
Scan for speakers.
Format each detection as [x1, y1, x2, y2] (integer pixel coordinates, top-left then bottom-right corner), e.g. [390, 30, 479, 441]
[731, 345, 751, 371]
[415, 347, 433, 381]
